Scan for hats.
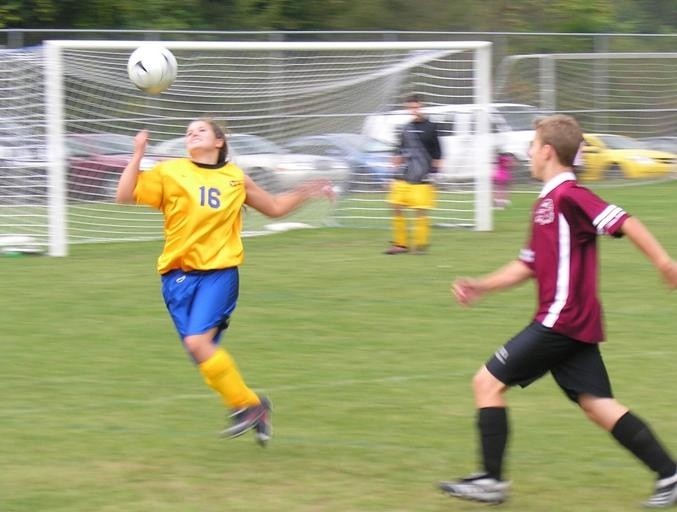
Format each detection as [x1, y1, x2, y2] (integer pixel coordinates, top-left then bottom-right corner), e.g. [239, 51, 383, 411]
[574, 132, 676, 181]
[286, 133, 412, 184]
[0, 124, 160, 204]
[155, 132, 349, 198]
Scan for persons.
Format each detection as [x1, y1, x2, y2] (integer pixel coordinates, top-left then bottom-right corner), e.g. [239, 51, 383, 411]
[382, 95, 445, 254]
[116, 119, 338, 448]
[438, 115, 677, 508]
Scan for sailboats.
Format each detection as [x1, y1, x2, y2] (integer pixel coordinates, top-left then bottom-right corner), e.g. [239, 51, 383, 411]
[127, 44, 177, 95]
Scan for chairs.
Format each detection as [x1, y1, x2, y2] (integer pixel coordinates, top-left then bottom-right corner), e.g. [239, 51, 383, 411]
[438, 472, 514, 502]
[641, 468, 677, 509]
[383, 245, 408, 255]
[219, 395, 273, 445]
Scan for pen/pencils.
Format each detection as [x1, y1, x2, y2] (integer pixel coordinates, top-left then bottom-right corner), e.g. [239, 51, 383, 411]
[368, 102, 581, 167]
